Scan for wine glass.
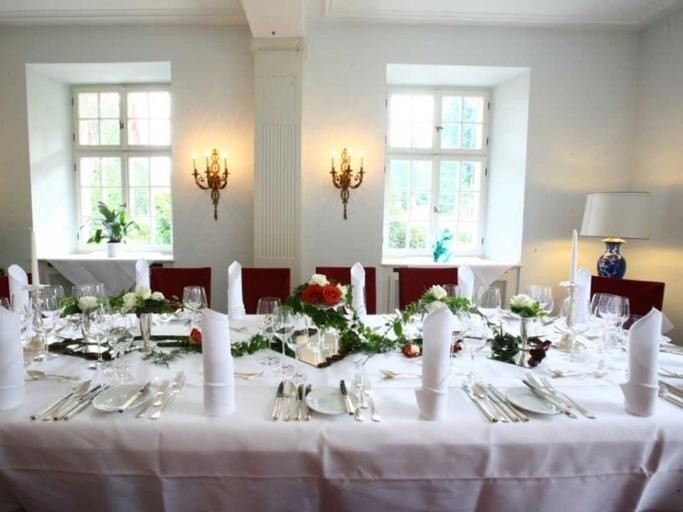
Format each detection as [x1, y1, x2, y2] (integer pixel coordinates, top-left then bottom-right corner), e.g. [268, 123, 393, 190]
[0, 284, 631, 380]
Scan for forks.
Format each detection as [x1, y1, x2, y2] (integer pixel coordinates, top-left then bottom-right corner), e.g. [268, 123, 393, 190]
[138, 373, 186, 422]
[352, 373, 382, 423]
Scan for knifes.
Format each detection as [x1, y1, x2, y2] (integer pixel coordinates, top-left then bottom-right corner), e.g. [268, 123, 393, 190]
[462, 371, 598, 421]
[338, 379, 353, 415]
[119, 379, 154, 414]
[270, 380, 315, 421]
[30, 374, 109, 423]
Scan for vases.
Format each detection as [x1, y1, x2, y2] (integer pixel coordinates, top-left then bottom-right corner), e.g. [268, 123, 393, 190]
[81, 311, 93, 342]
[140, 314, 155, 352]
[513, 317, 535, 367]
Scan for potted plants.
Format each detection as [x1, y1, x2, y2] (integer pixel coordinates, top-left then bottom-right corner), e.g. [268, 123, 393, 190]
[80, 201, 141, 256]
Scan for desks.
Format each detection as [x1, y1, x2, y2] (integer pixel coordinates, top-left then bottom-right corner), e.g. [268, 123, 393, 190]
[37, 258, 175, 298]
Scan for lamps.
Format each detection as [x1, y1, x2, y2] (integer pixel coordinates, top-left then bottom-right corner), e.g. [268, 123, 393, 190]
[329, 149, 365, 219]
[580, 193, 652, 279]
[191, 147, 231, 220]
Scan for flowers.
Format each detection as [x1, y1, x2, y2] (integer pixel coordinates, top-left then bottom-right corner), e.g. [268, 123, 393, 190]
[511, 294, 548, 318]
[420, 286, 457, 306]
[58, 289, 125, 320]
[121, 286, 170, 314]
[283, 273, 354, 330]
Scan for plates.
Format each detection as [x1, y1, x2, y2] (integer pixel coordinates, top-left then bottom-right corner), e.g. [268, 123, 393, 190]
[305, 386, 360, 414]
[650, 334, 683, 378]
[92, 383, 155, 412]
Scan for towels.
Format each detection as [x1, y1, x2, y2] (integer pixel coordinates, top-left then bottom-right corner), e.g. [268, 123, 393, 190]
[227, 262, 246, 331]
[200, 308, 234, 418]
[458, 263, 474, 302]
[350, 262, 365, 316]
[415, 307, 453, 420]
[135, 259, 150, 288]
[7, 265, 31, 312]
[619, 307, 663, 416]
[1, 305, 26, 409]
[574, 270, 591, 323]
